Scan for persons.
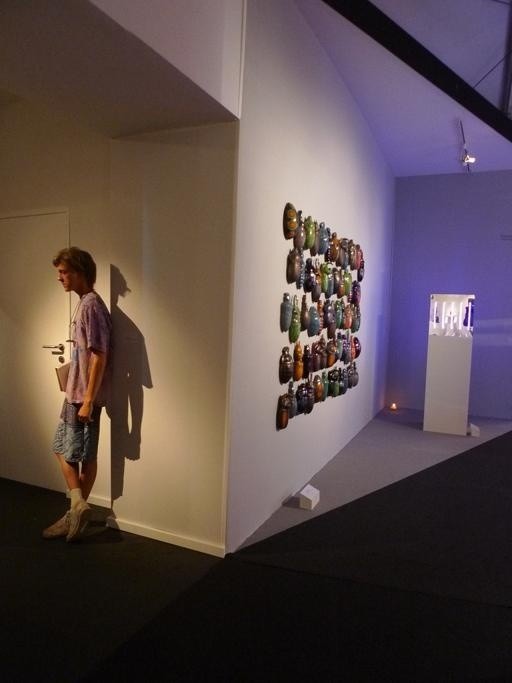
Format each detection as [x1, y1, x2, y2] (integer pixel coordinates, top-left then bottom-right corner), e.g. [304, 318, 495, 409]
[41, 243, 113, 544]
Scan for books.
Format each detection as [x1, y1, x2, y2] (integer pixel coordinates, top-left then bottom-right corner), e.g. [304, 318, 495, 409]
[56, 360, 71, 392]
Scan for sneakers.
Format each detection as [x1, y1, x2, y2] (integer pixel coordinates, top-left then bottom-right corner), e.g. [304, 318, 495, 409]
[67, 500, 91, 542]
[41, 505, 86, 539]
